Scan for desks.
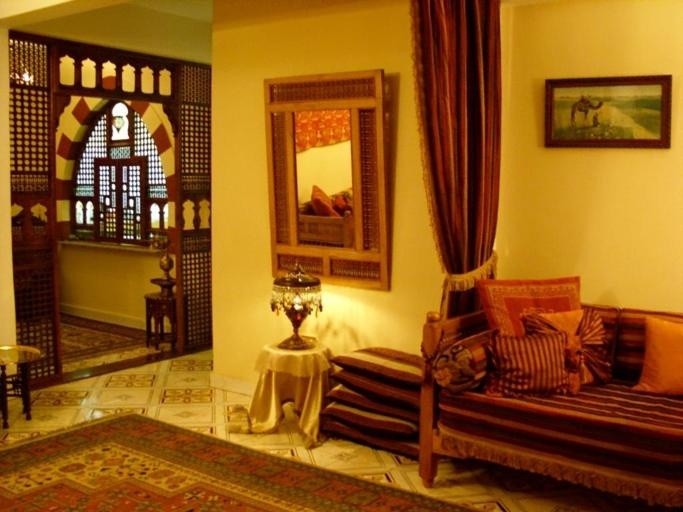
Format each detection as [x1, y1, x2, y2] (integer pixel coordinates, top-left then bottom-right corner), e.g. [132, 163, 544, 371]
[261, 340, 327, 424]
[0, 343, 48, 429]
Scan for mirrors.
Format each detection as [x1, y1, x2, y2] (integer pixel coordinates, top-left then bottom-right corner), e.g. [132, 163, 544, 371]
[262, 70, 390, 293]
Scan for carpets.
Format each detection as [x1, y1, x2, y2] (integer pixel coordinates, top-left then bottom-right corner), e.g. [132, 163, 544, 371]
[1, 412, 478, 512]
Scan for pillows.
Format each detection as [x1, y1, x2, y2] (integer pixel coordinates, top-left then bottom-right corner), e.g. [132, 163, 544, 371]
[320, 346, 426, 462]
[311, 184, 352, 217]
[429, 274, 683, 401]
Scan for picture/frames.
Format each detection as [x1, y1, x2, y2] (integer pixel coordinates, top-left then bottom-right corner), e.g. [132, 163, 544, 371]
[544, 73, 673, 150]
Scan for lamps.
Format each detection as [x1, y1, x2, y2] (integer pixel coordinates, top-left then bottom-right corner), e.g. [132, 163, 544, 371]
[270, 259, 323, 350]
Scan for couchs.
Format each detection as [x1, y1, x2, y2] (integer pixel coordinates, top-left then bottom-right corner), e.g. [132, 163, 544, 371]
[295, 186, 355, 248]
[416, 302, 682, 507]
